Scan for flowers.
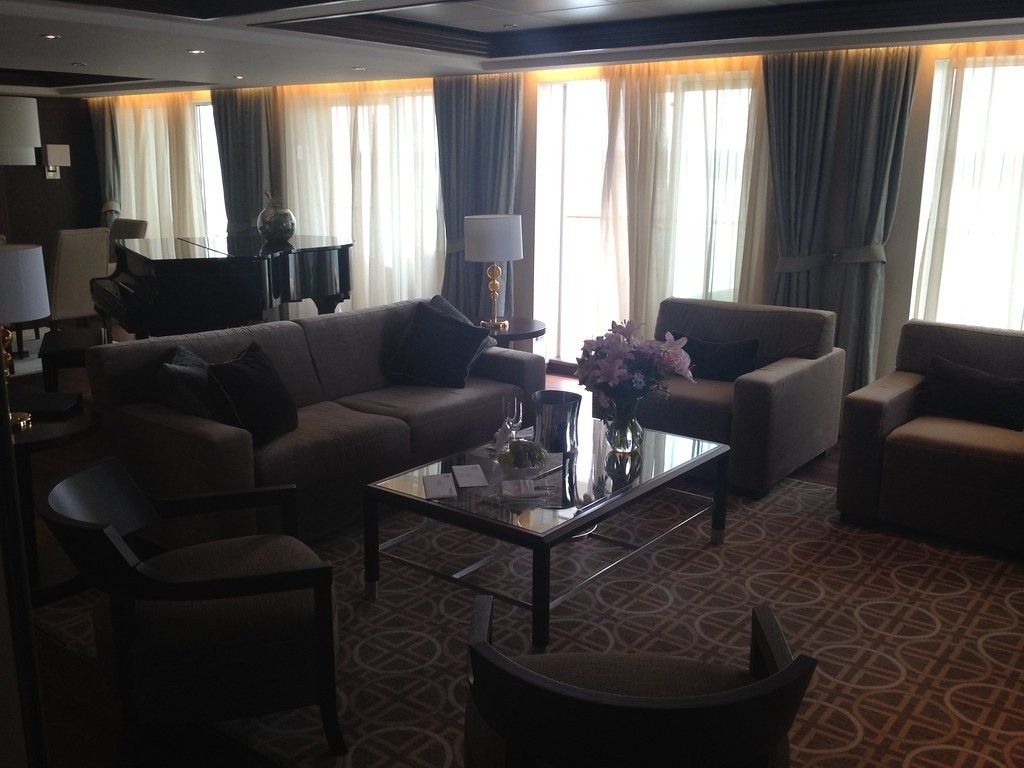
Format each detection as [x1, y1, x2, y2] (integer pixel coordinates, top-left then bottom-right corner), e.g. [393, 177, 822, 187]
[259, 191, 294, 239]
[573, 317, 693, 449]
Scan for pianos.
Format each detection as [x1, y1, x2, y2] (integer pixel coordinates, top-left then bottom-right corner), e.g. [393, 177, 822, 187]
[88, 234, 355, 341]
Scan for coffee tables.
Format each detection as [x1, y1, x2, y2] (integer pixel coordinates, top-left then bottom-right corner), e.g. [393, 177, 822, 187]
[362, 417, 731, 648]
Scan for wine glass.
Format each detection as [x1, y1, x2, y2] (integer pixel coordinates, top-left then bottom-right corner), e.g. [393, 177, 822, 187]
[503, 394, 523, 451]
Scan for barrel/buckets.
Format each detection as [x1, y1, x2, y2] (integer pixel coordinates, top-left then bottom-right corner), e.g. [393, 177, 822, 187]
[532, 389, 582, 453]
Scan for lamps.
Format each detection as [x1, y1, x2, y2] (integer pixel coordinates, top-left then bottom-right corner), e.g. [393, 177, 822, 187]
[0, 96, 44, 166]
[0, 243, 51, 432]
[464, 214, 523, 328]
[42, 144, 71, 180]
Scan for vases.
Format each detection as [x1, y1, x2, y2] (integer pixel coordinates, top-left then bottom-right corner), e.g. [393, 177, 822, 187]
[256, 208, 297, 242]
[603, 395, 647, 451]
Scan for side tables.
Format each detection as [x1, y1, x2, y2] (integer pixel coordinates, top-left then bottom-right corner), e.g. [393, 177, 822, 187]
[10, 394, 101, 600]
[476, 316, 546, 346]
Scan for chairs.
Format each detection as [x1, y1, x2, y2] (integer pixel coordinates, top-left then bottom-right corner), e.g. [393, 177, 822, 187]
[465, 591, 820, 768]
[44, 454, 347, 768]
[53, 219, 148, 349]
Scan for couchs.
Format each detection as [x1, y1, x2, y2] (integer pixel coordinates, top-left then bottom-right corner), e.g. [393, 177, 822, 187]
[84, 295, 546, 546]
[837, 324, 1024, 556]
[587, 298, 844, 495]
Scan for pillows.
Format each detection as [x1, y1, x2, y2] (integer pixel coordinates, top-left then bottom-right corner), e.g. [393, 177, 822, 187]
[925, 354, 1024, 431]
[158, 343, 240, 429]
[673, 330, 760, 382]
[205, 340, 298, 451]
[384, 301, 490, 391]
[394, 295, 497, 368]
